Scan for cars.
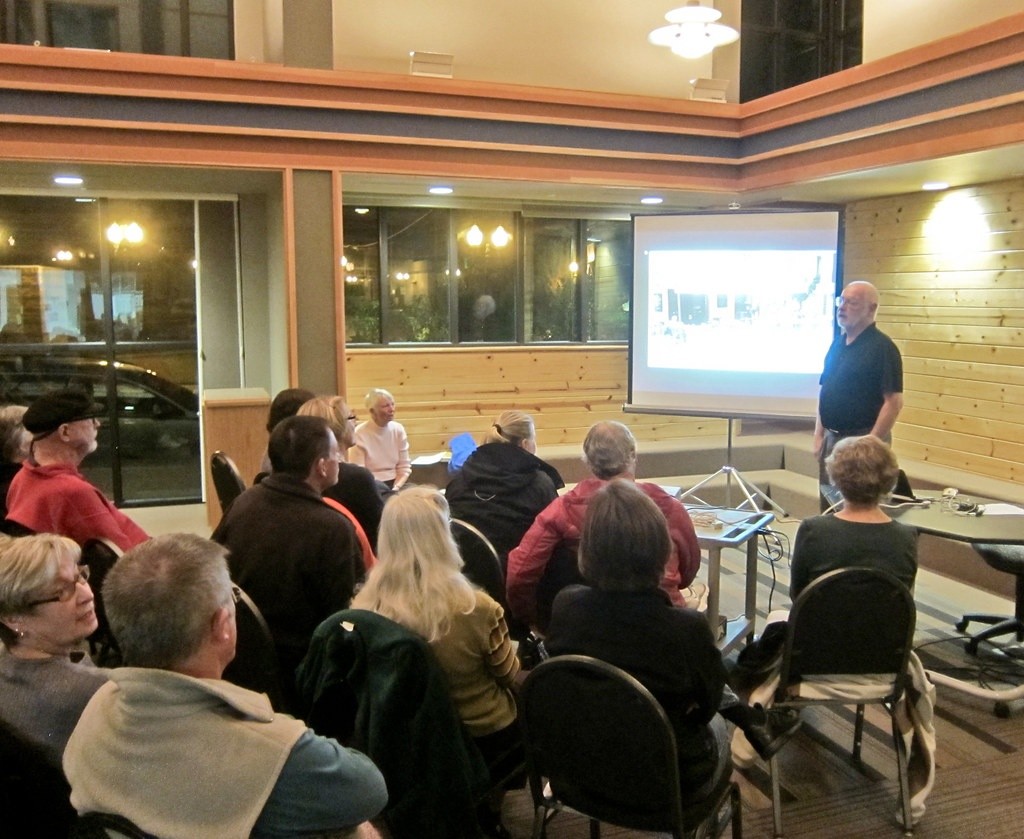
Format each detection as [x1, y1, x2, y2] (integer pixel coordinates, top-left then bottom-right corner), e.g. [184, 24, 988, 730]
[0, 355, 199, 454]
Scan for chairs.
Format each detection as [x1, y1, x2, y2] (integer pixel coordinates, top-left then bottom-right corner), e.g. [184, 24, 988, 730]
[0, 448, 1024, 839]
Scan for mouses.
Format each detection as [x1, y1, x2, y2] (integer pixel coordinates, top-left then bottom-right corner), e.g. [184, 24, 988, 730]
[942, 487, 958, 499]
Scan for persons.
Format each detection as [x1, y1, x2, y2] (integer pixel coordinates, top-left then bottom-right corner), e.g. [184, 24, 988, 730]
[209, 416, 376, 666]
[442, 409, 566, 578]
[813, 280, 905, 522]
[260, 388, 316, 475]
[787, 434, 917, 610]
[354, 387, 417, 503]
[295, 395, 385, 555]
[503, 419, 710, 628]
[351, 488, 522, 776]
[61, 534, 388, 839]
[0, 532, 113, 839]
[0, 405, 34, 529]
[544, 476, 804, 818]
[5, 388, 153, 563]
[473, 294, 499, 341]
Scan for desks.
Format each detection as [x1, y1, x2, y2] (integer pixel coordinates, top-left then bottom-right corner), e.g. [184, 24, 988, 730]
[871, 490, 1024, 661]
[675, 505, 773, 656]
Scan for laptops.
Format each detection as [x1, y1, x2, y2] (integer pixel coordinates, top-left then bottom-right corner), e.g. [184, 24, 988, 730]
[891, 469, 935, 503]
